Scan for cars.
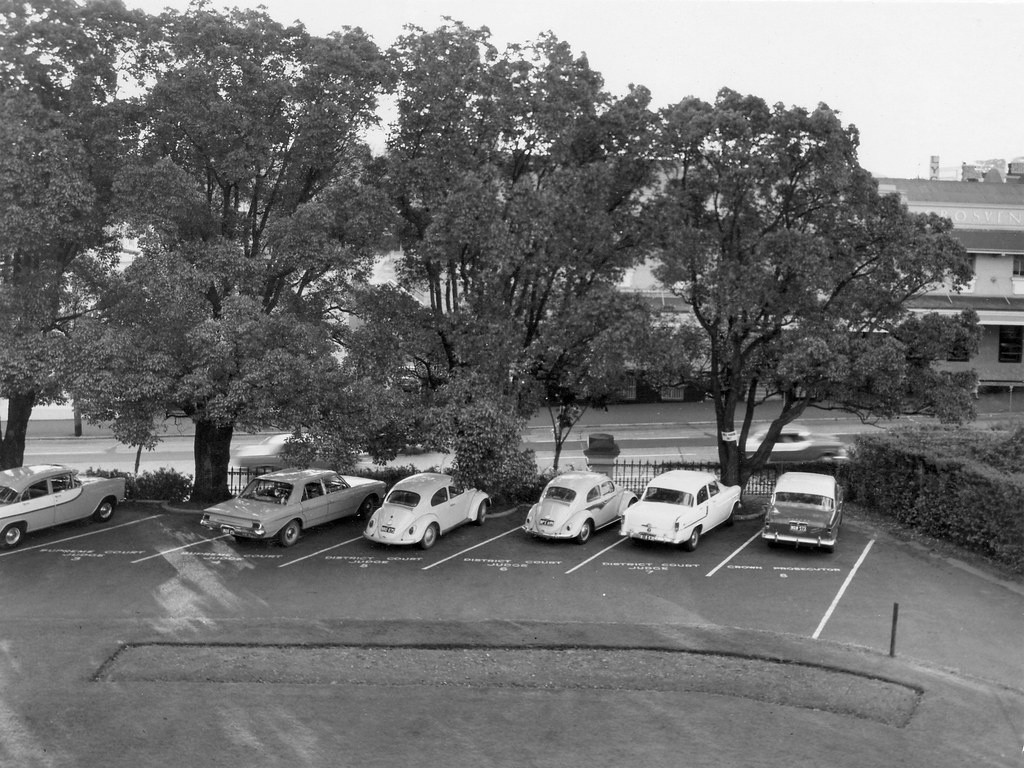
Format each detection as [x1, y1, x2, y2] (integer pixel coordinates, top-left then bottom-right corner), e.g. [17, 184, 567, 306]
[0, 465, 125, 549]
[762, 470, 844, 553]
[199, 466, 388, 548]
[234, 433, 360, 472]
[736, 422, 844, 462]
[363, 473, 493, 550]
[618, 470, 742, 552]
[522, 472, 639, 545]
